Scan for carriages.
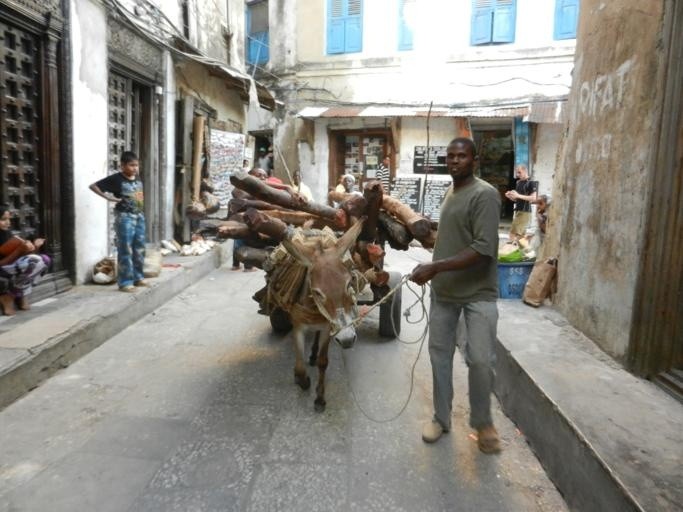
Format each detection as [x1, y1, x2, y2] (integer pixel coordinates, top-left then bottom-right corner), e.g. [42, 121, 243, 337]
[253, 216, 403, 413]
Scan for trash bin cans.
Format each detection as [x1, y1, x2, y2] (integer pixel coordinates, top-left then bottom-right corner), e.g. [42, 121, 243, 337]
[497, 262, 534, 299]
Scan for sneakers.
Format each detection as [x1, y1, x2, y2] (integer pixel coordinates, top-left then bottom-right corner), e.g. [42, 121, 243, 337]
[478, 424, 501, 454]
[422, 420, 444, 443]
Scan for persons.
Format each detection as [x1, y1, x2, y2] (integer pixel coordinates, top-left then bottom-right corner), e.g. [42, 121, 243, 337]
[333, 171, 363, 209]
[529, 193, 549, 246]
[0, 206, 51, 317]
[504, 162, 538, 250]
[405, 135, 503, 457]
[230, 167, 268, 275]
[286, 169, 316, 203]
[87, 149, 152, 294]
[375, 154, 392, 198]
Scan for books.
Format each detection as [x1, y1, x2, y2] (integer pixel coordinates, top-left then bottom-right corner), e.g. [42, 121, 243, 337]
[0, 234, 47, 258]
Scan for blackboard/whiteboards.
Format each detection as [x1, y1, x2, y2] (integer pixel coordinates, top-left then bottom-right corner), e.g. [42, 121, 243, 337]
[393, 178, 452, 219]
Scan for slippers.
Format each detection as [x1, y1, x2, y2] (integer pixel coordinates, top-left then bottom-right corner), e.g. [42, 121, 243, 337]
[119, 278, 150, 292]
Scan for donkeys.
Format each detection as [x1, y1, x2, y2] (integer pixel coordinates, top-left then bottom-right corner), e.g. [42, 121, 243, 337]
[268, 215, 369, 413]
